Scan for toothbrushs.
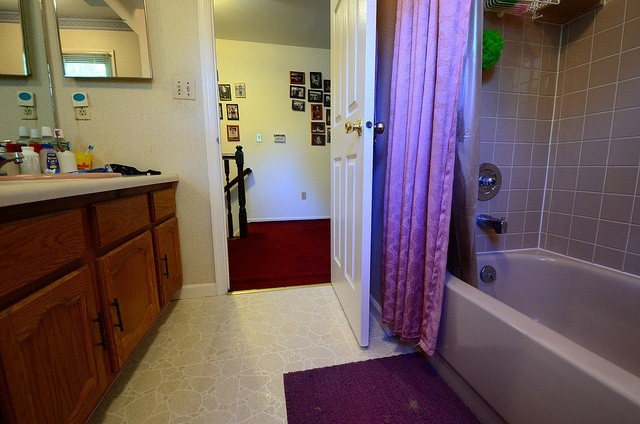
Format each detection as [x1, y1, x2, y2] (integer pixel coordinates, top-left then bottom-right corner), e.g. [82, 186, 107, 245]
[85, 144, 94, 154]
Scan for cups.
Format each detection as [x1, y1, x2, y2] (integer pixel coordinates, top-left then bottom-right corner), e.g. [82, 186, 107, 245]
[74, 151, 93, 171]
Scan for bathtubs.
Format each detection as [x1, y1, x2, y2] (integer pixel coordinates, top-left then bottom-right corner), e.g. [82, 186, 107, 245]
[432, 247, 637, 424]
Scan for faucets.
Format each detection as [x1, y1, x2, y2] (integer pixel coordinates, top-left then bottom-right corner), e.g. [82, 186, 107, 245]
[0, 146, 23, 169]
[477, 214, 508, 235]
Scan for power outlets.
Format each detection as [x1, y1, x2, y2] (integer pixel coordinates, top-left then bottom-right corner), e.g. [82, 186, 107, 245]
[21, 106, 37, 120]
[74, 107, 90, 120]
[172, 79, 196, 100]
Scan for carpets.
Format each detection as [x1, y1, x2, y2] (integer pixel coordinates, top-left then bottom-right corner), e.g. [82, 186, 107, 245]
[283, 352, 482, 424]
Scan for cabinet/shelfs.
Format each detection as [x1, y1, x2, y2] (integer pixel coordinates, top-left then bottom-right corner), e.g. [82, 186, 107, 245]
[149, 181, 183, 315]
[1, 186, 161, 424]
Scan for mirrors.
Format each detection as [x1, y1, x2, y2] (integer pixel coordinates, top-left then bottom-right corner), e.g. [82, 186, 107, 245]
[0, 0, 57, 145]
[0, 0, 31, 77]
[54, 0, 152, 80]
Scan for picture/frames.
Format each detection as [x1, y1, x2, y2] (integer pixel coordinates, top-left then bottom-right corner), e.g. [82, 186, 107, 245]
[291, 99, 306, 113]
[290, 71, 305, 84]
[323, 79, 330, 93]
[219, 103, 223, 120]
[235, 83, 246, 98]
[310, 122, 325, 133]
[310, 72, 322, 89]
[327, 127, 331, 143]
[310, 104, 323, 120]
[308, 89, 322, 103]
[226, 104, 239, 120]
[290, 85, 305, 99]
[324, 94, 330, 107]
[310, 133, 326, 146]
[218, 84, 231, 101]
[227, 125, 240, 141]
[326, 109, 330, 125]
